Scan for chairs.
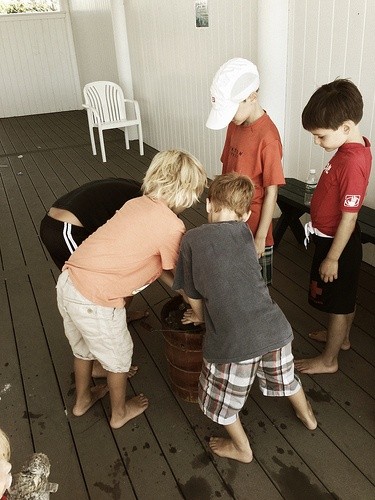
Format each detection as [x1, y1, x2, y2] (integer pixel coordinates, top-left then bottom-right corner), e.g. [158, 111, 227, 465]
[83, 80, 144, 161]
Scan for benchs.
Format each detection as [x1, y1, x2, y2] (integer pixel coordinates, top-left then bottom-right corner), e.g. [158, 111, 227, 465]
[273, 178, 375, 279]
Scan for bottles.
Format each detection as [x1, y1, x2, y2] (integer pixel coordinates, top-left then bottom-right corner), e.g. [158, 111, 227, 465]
[304, 169, 318, 206]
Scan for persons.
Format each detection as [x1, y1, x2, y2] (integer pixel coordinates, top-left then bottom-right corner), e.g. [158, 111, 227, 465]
[205, 57, 286, 287]
[172, 174, 319, 463]
[293, 79, 373, 375]
[40, 177, 192, 380]
[53, 150, 206, 429]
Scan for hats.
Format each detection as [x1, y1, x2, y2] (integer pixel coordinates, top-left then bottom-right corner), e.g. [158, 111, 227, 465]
[205, 57, 259, 130]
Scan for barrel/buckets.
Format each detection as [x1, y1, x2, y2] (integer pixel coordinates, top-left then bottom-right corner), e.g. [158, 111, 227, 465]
[140, 294, 202, 403]
